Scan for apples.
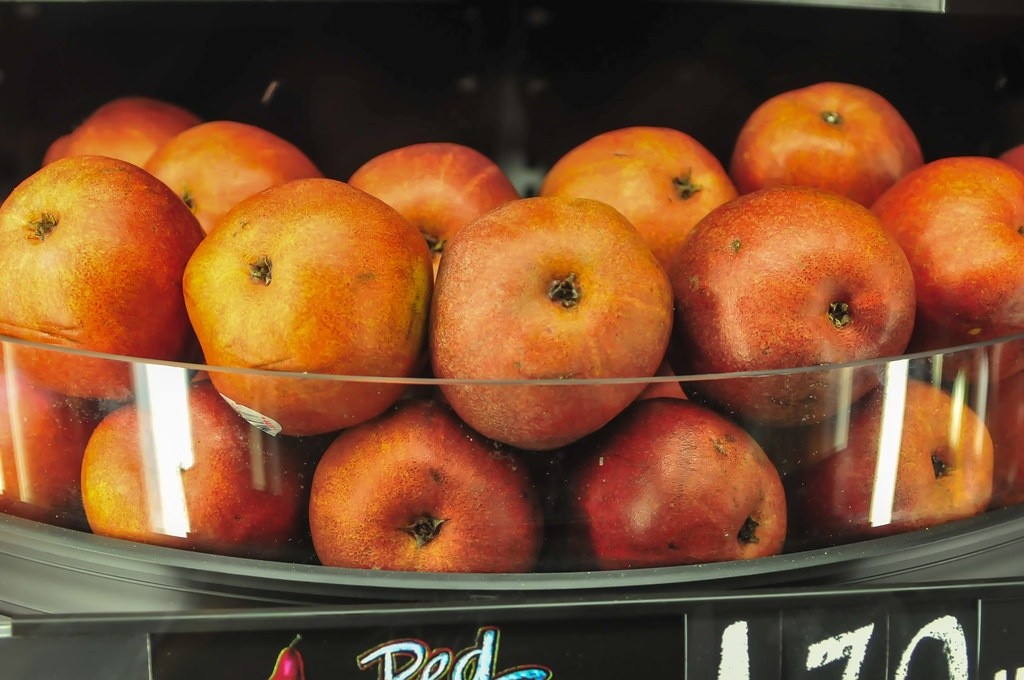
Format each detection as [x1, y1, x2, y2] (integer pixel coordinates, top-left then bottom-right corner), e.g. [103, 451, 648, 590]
[0, 81, 1024, 571]
